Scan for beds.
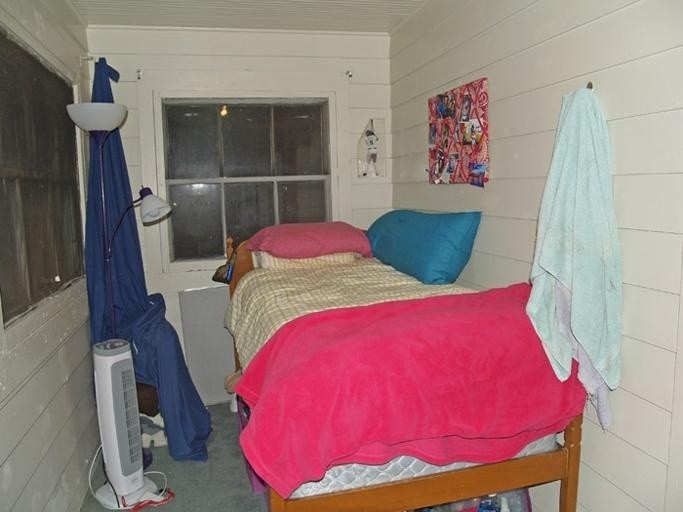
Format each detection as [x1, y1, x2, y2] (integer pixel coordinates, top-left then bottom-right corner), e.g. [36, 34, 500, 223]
[213, 235, 585, 510]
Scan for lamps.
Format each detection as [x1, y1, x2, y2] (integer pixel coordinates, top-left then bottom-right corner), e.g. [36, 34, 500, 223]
[66, 103, 173, 471]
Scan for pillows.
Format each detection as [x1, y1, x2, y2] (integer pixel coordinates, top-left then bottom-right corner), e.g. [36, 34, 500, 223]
[242, 221, 372, 259]
[366, 208, 483, 285]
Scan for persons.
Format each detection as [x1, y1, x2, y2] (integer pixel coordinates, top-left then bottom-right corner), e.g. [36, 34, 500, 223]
[363, 128, 381, 175]
